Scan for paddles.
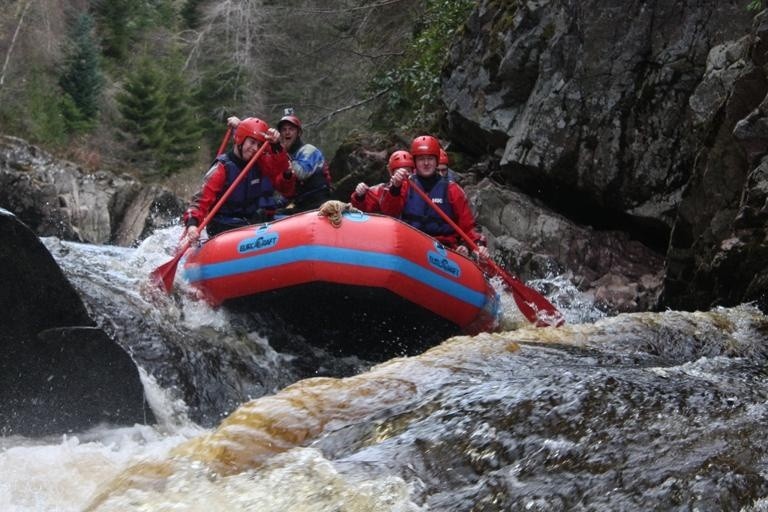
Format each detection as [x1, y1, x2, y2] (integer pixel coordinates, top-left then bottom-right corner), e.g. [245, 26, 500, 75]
[407, 178, 567, 327]
[149, 130, 275, 295]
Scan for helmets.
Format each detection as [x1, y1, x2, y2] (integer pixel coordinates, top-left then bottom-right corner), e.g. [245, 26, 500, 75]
[235, 116, 269, 148]
[411, 136, 449, 165]
[388, 151, 415, 169]
[277, 115, 301, 129]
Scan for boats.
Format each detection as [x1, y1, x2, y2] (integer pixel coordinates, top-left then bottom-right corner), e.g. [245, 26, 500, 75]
[182, 200, 501, 337]
